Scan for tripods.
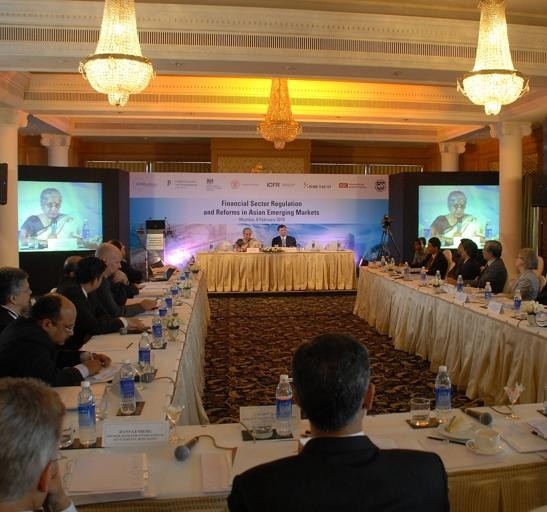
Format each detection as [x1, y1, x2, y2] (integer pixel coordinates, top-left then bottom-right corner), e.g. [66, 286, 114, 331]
[378, 229, 401, 258]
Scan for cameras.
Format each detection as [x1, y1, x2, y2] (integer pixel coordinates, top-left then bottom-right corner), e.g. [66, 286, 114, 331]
[382, 214, 393, 229]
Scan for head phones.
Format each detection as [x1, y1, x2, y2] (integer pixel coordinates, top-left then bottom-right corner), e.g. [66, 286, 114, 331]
[420, 238, 424, 248]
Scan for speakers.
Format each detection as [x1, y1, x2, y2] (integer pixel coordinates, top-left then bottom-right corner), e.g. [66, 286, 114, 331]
[531, 172, 546, 208]
[0, 163, 8, 205]
[146, 220, 165, 234]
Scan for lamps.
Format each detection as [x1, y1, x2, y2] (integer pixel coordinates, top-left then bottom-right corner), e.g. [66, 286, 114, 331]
[455, 1, 530, 120]
[75, 1, 153, 108]
[253, 78, 303, 153]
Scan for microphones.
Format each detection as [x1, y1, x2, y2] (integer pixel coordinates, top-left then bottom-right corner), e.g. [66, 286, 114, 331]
[107, 372, 152, 383]
[51, 217, 57, 234]
[457, 218, 462, 232]
[175, 436, 199, 461]
[461, 406, 492, 425]
[120, 328, 152, 335]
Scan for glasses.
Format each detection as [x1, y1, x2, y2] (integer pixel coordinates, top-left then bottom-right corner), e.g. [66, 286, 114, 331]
[451, 204, 465, 209]
[64, 327, 74, 337]
[51, 450, 66, 465]
[45, 202, 60, 209]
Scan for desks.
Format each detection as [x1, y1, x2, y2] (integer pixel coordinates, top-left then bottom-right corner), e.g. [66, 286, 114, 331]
[197, 249, 356, 292]
[353, 264, 546, 406]
[49, 280, 211, 426]
[55, 406, 547, 511]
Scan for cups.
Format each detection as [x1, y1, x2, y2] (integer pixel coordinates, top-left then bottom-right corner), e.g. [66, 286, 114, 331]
[53, 415, 73, 450]
[250, 411, 275, 439]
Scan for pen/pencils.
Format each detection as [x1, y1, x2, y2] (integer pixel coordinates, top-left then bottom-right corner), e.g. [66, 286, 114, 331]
[305, 431, 311, 434]
[530, 430, 544, 439]
[390, 275, 400, 276]
[426, 436, 466, 445]
[88, 352, 93, 361]
[155, 297, 159, 301]
[395, 276, 404, 279]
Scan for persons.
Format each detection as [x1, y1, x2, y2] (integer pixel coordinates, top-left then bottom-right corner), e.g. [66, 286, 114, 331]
[20, 187, 82, 246]
[430, 191, 484, 243]
[226, 332, 450, 512]
[410, 236, 540, 302]
[272, 224, 297, 248]
[233, 228, 257, 251]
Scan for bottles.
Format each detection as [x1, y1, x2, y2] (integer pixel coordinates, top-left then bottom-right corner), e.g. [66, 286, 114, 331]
[371, 251, 547, 455]
[206, 239, 346, 253]
[75, 255, 197, 449]
[275, 372, 293, 439]
